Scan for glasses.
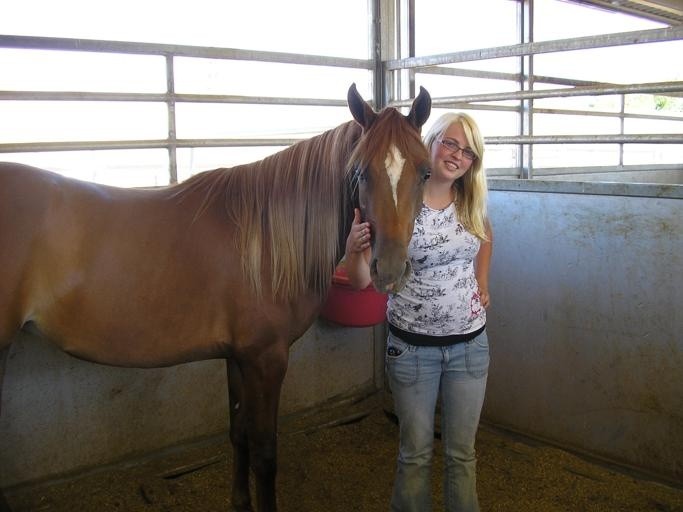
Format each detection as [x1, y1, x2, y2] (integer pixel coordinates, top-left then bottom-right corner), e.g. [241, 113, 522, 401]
[437, 139, 477, 160]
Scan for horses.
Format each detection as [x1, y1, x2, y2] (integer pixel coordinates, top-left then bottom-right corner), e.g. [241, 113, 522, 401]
[0, 81, 433, 511]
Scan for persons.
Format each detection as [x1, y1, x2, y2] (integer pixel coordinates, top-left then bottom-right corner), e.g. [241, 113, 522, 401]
[344, 111, 492, 511]
